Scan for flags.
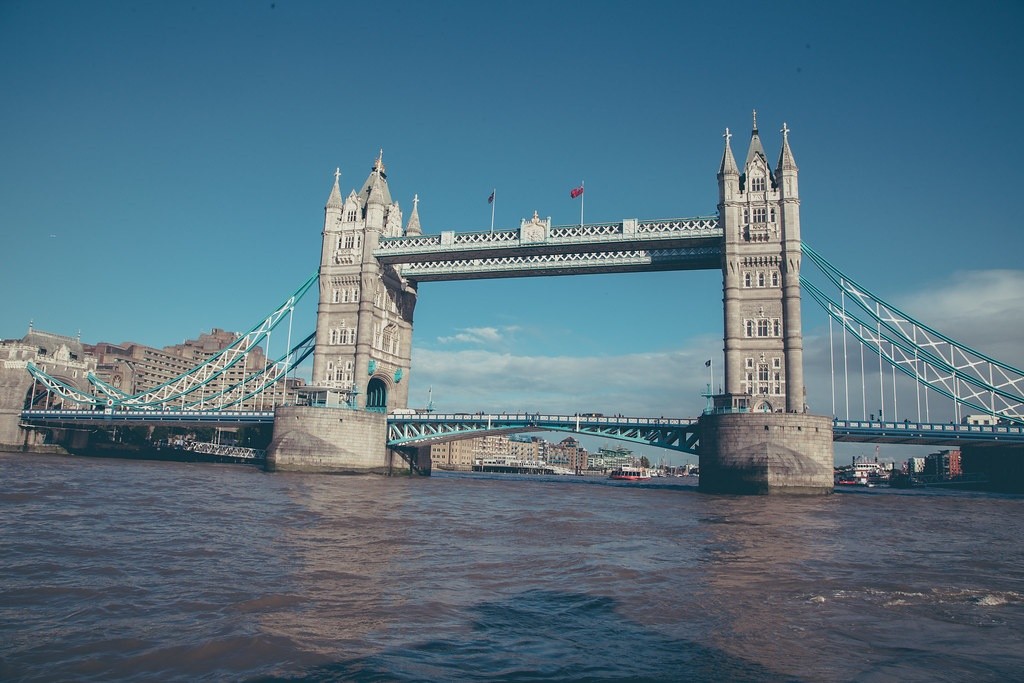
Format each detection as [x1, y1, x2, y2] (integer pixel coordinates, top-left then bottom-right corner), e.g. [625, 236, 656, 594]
[705, 360, 711, 367]
[488, 192, 494, 203]
[571, 186, 583, 198]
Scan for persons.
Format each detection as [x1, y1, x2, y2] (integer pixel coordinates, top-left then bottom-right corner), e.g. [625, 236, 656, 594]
[904, 419, 911, 422]
[575, 413, 597, 416]
[950, 420, 953, 424]
[305, 396, 312, 406]
[614, 413, 624, 417]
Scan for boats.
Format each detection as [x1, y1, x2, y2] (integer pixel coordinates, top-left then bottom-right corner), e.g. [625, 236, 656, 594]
[834, 453, 926, 488]
[936, 472, 991, 490]
[610, 466, 652, 481]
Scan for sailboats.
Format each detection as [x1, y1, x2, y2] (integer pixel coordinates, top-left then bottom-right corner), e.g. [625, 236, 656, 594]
[651, 456, 699, 477]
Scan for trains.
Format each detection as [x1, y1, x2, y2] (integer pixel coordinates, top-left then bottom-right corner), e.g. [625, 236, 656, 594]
[961, 414, 1024, 427]
